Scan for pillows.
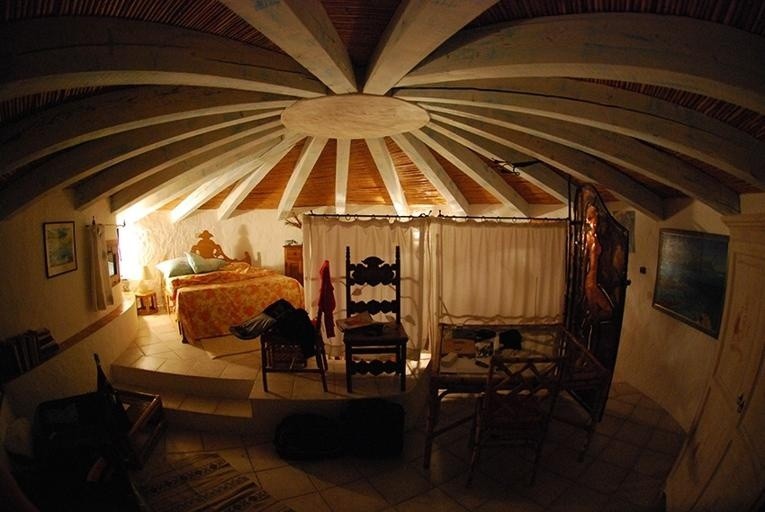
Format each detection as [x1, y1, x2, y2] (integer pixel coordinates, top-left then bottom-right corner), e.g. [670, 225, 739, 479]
[153, 251, 224, 278]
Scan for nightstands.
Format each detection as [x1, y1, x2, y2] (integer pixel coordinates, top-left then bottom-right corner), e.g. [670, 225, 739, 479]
[282, 246, 303, 280]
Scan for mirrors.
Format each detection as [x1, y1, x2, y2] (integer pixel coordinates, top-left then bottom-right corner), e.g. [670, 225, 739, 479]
[105, 240, 120, 288]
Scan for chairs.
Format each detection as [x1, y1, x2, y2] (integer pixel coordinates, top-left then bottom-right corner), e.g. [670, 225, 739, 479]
[259, 259, 334, 392]
[460, 354, 566, 489]
[343, 244, 409, 394]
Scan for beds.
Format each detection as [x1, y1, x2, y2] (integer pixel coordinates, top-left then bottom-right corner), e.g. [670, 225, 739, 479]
[171, 229, 303, 343]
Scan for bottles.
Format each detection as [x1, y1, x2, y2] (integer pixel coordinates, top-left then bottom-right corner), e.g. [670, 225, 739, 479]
[93, 352, 131, 430]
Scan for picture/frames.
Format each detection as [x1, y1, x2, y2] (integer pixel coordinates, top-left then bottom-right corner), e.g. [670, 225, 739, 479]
[650, 227, 731, 340]
[44, 220, 78, 278]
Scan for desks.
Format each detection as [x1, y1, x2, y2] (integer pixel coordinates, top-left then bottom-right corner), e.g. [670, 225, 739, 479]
[136, 290, 159, 316]
[420, 320, 608, 470]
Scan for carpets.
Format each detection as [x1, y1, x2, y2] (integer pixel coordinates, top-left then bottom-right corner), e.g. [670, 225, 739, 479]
[198, 333, 259, 358]
[140, 450, 301, 512]
